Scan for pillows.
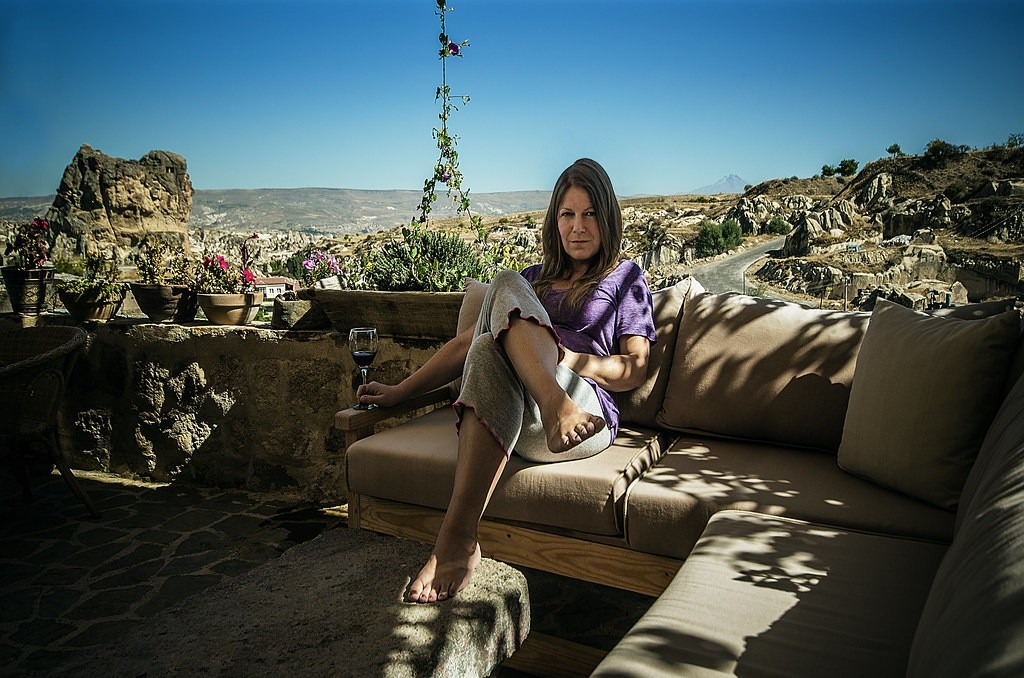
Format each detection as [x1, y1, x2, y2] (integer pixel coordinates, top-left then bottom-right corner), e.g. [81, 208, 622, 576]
[456, 276, 1024, 514]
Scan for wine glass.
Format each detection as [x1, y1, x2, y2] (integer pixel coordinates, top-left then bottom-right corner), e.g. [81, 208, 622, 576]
[348, 327, 379, 410]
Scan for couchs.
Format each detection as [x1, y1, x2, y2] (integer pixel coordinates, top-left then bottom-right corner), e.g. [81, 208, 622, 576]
[335, 395, 1024, 678]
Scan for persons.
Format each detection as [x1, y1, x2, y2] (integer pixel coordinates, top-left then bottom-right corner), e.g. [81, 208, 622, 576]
[356, 158, 656, 602]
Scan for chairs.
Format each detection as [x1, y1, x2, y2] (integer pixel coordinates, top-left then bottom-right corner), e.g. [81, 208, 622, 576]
[0, 324, 91, 521]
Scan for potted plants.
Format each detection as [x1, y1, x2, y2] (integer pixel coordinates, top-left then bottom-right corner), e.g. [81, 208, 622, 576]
[0, 218, 507, 340]
